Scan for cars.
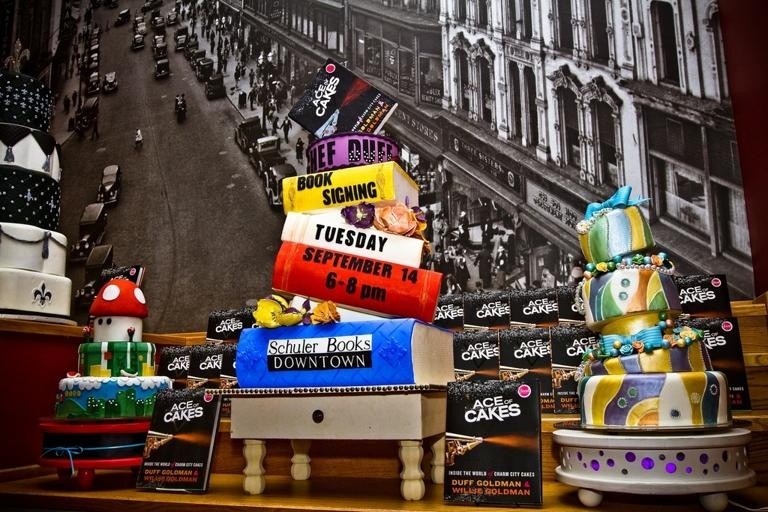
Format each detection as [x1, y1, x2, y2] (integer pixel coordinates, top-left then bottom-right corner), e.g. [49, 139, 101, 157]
[73, 246, 113, 303]
[232, 115, 299, 210]
[98, 165, 122, 207]
[68, 203, 110, 260]
[75, 2, 225, 143]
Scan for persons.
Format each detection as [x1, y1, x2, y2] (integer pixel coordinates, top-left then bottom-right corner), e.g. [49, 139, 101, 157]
[409, 167, 541, 295]
[61, 0, 303, 163]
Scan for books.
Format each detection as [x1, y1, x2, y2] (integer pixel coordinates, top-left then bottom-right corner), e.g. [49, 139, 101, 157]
[673, 272, 752, 411]
[433, 272, 606, 506]
[288, 57, 397, 139]
[88, 264, 145, 328]
[134, 306, 255, 493]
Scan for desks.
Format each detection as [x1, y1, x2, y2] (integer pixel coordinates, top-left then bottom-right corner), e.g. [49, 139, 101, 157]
[205, 383, 448, 500]
